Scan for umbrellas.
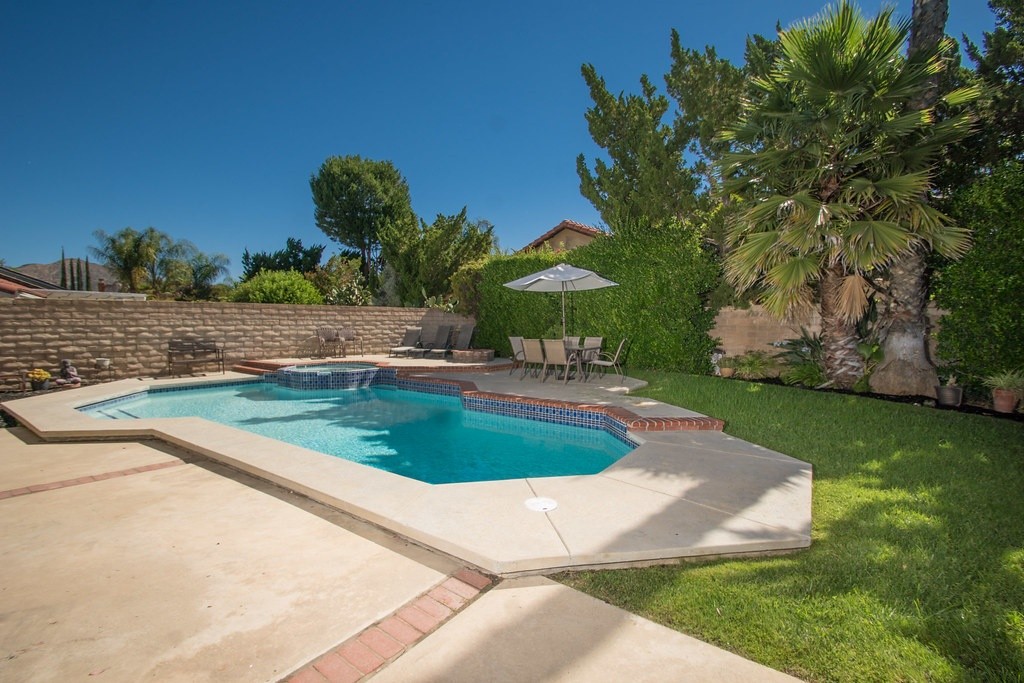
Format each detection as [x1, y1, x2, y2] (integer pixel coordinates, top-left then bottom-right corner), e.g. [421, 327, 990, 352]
[502, 263, 619, 339]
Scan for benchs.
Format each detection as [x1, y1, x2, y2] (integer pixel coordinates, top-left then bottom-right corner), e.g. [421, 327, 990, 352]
[166, 340, 225, 379]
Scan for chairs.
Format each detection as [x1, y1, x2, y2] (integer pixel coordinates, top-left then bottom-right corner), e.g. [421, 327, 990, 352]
[317, 327, 363, 360]
[506, 337, 538, 377]
[431, 324, 476, 359]
[589, 338, 626, 383]
[547, 336, 580, 375]
[389, 327, 422, 357]
[542, 340, 577, 384]
[520, 339, 552, 381]
[408, 325, 452, 358]
[573, 337, 603, 377]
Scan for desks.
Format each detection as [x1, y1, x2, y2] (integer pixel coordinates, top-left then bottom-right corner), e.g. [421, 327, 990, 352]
[542, 345, 601, 378]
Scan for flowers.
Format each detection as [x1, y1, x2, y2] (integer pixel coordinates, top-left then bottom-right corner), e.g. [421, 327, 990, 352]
[29, 369, 50, 381]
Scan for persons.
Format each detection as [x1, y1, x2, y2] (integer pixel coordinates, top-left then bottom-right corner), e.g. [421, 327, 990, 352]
[56, 359, 81, 384]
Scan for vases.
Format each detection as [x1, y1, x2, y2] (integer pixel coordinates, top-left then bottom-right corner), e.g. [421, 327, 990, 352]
[31, 380, 49, 390]
[96, 358, 110, 369]
